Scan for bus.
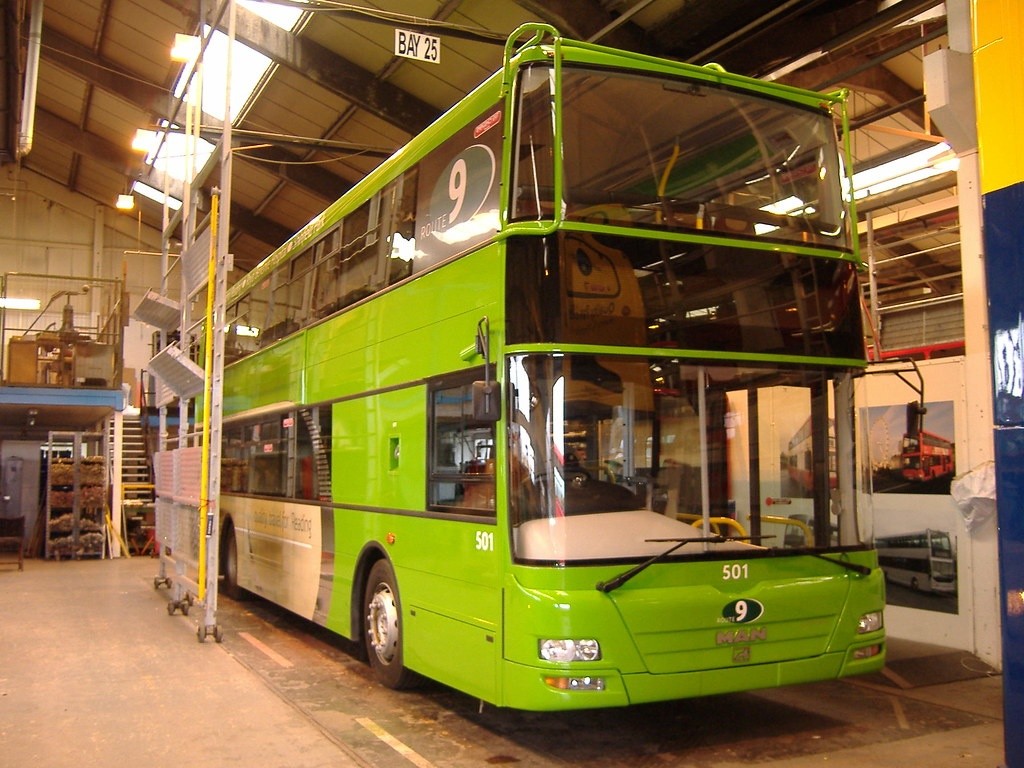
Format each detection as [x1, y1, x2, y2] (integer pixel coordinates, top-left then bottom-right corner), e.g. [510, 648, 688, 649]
[786, 413, 837, 490]
[901, 429, 954, 483]
[783, 513, 839, 549]
[873, 528, 957, 596]
[196, 23, 890, 711]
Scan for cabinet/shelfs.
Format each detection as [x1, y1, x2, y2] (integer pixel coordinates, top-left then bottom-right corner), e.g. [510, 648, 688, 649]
[7, 335, 39, 381]
[71, 343, 116, 387]
[47, 430, 108, 559]
